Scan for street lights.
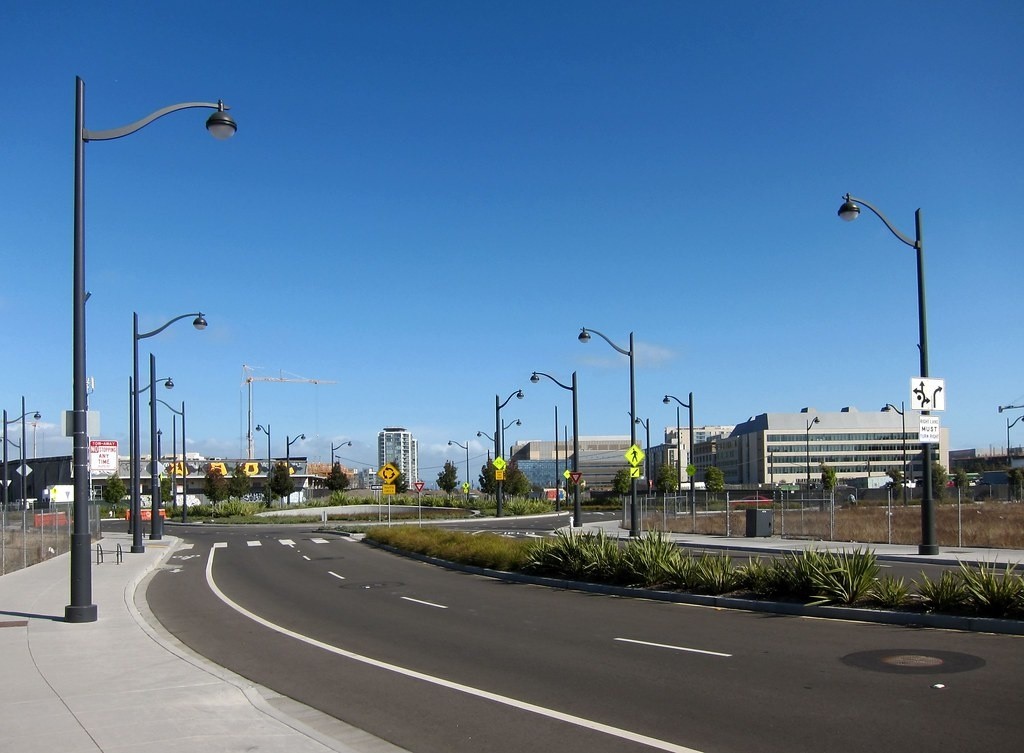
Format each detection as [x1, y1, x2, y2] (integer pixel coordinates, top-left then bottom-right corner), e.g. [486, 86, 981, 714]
[494, 388, 524, 518]
[0, 435, 23, 512]
[502, 418, 522, 504]
[476, 431, 498, 502]
[63, 71, 240, 623]
[148, 396, 188, 524]
[331, 440, 353, 473]
[530, 369, 582, 528]
[285, 432, 306, 505]
[1006, 415, 1024, 463]
[662, 391, 697, 517]
[448, 440, 470, 502]
[577, 326, 639, 537]
[255, 424, 271, 508]
[128, 375, 175, 534]
[837, 193, 940, 556]
[2, 409, 42, 512]
[806, 416, 820, 507]
[880, 400, 907, 507]
[130, 309, 209, 554]
[635, 416, 652, 511]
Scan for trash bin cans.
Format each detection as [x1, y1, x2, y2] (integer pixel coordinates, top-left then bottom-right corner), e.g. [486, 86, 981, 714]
[746, 508, 773, 538]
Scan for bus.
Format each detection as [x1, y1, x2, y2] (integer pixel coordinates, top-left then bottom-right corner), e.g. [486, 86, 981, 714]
[937, 472, 983, 488]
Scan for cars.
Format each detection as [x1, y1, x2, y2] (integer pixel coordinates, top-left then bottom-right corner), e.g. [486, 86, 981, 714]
[900, 478, 919, 489]
[729, 494, 774, 509]
